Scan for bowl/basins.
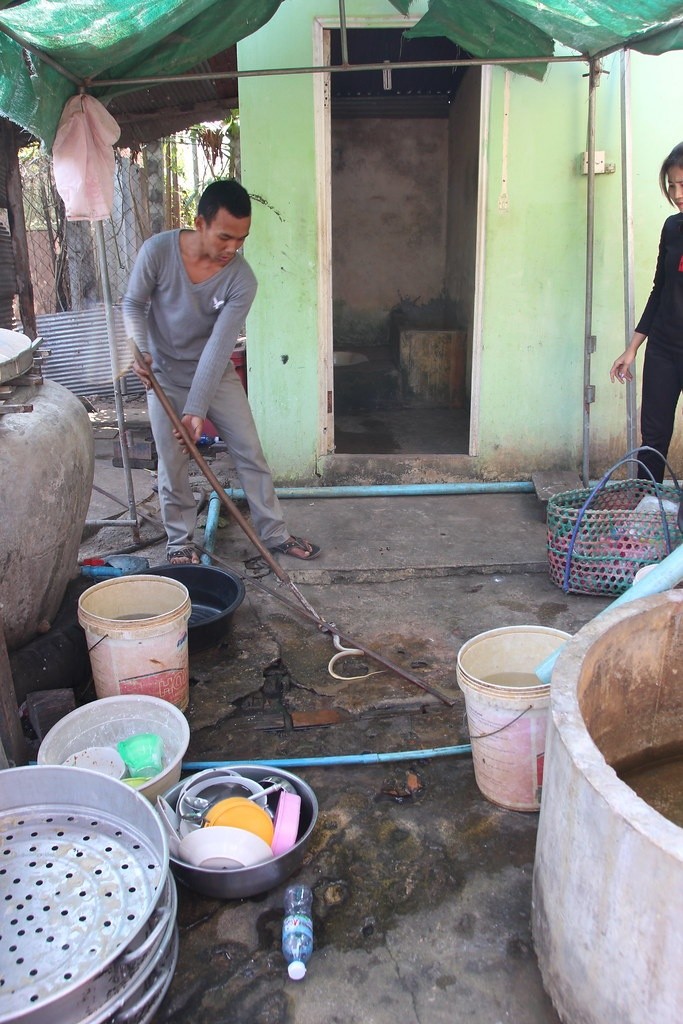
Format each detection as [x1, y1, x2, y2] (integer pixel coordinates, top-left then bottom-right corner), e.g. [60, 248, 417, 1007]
[131, 562, 246, 654]
[154, 762, 319, 899]
[36, 694, 190, 806]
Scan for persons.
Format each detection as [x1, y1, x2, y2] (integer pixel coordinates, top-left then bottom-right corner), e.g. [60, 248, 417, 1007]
[609, 142, 683, 483]
[124, 180, 322, 567]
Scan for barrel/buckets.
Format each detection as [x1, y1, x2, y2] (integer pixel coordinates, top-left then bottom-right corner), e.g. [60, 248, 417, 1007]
[455, 625, 573, 812]
[77, 573, 190, 713]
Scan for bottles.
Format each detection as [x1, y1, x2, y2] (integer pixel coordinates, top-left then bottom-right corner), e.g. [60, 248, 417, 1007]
[282, 883, 314, 980]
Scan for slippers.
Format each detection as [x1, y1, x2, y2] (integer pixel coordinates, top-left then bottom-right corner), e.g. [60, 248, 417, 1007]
[166, 547, 200, 564]
[273, 536, 322, 559]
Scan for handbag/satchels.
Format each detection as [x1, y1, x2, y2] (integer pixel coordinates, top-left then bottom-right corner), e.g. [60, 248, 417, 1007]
[547, 444, 683, 597]
